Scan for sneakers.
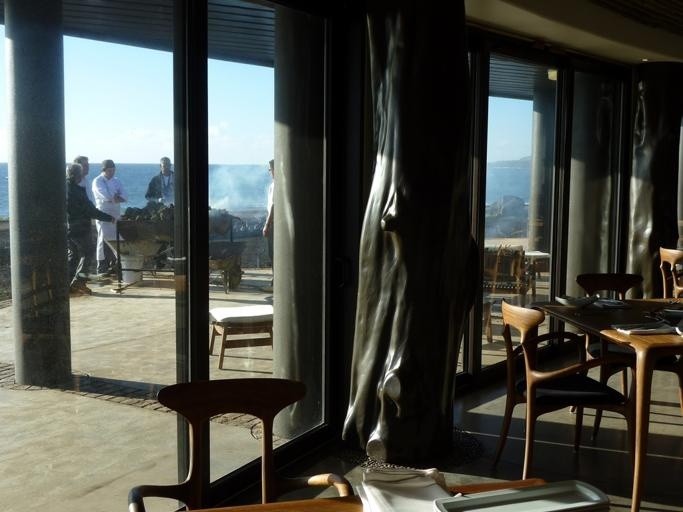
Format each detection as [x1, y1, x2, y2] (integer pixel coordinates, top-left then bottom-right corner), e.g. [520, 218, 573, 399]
[97, 273, 110, 277]
[261, 285, 273, 292]
[69, 279, 91, 297]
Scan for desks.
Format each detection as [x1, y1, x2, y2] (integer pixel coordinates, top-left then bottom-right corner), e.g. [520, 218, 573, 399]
[180, 475, 611, 512]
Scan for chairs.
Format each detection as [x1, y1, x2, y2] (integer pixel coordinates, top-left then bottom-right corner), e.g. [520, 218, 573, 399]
[127, 378, 365, 512]
[479, 245, 681, 511]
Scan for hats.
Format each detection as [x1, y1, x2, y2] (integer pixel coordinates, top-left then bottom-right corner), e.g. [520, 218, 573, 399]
[102, 160, 115, 169]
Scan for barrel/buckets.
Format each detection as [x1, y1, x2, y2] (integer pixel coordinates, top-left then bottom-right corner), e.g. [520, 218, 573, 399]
[120, 254, 144, 284]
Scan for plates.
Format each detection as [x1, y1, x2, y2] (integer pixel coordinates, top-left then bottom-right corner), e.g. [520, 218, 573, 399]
[555, 293, 600, 308]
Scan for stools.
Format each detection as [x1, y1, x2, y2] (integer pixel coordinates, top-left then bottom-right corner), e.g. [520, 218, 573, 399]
[207, 304, 274, 371]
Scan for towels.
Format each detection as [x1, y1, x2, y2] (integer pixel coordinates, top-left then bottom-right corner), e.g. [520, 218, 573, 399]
[357, 466, 461, 512]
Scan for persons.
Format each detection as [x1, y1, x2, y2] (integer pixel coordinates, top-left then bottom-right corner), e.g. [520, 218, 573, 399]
[67, 155, 128, 295]
[144, 157, 175, 206]
[259, 159, 274, 292]
